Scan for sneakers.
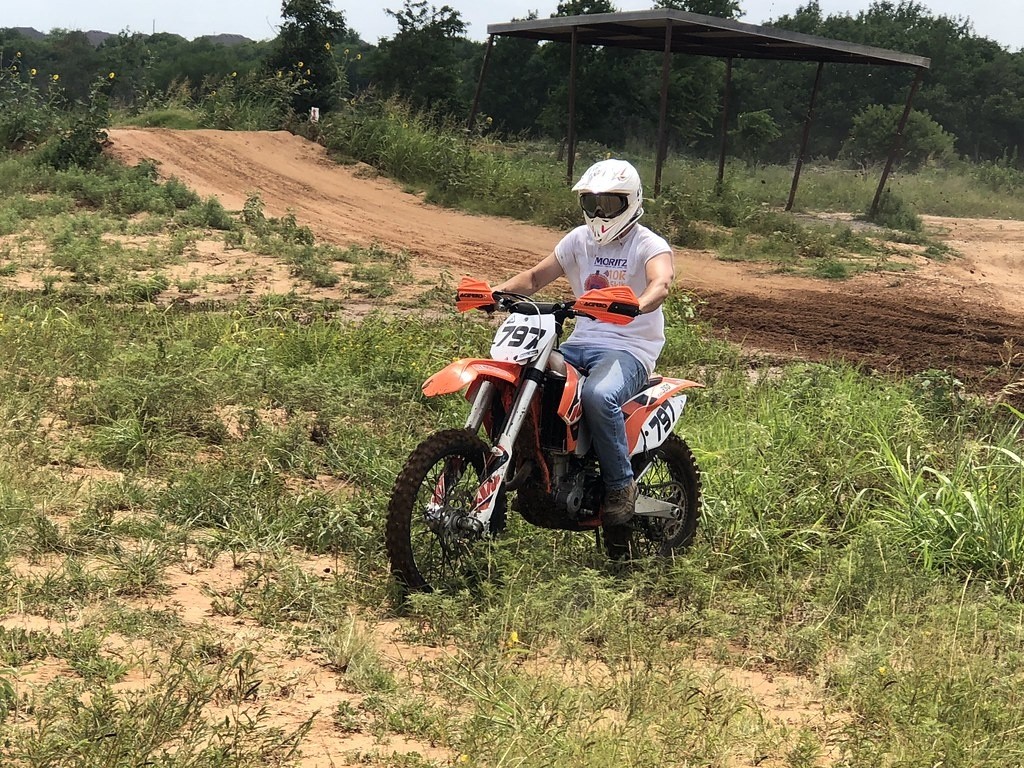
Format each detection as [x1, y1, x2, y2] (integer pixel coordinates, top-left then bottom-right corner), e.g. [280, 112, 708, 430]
[510, 496, 519, 510]
[601, 478, 640, 526]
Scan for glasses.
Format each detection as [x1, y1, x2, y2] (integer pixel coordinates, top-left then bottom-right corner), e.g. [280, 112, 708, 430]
[578, 192, 629, 219]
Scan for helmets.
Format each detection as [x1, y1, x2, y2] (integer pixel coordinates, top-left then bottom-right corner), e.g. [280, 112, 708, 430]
[571, 159, 644, 247]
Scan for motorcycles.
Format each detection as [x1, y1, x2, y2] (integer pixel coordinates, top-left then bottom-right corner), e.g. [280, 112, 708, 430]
[383, 277, 706, 604]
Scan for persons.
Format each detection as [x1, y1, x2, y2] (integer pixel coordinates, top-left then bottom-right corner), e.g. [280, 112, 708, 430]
[489, 158, 674, 527]
